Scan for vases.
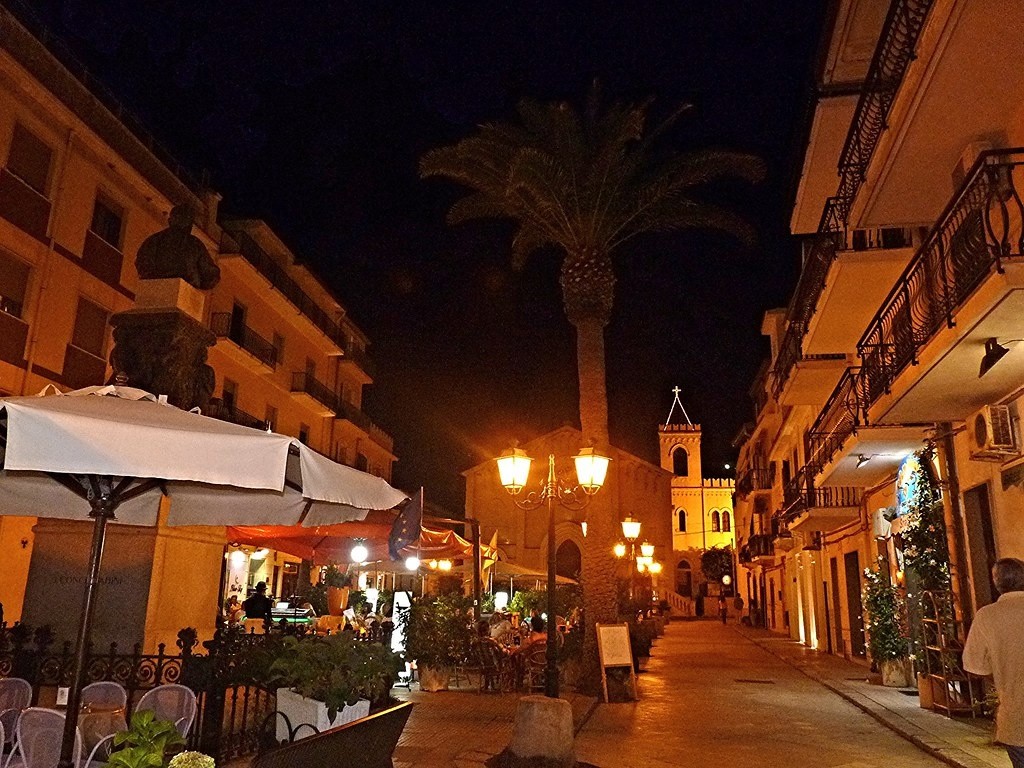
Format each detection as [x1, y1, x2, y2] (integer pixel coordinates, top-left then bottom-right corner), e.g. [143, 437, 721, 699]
[916, 671, 948, 709]
[880, 655, 910, 687]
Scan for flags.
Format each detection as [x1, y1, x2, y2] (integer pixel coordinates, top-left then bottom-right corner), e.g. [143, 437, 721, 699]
[389, 487, 422, 563]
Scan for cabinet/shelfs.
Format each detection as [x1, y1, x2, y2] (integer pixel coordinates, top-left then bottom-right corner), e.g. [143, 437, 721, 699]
[921, 589, 976, 720]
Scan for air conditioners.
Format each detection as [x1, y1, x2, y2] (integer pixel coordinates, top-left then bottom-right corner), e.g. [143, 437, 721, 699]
[965, 405, 1015, 455]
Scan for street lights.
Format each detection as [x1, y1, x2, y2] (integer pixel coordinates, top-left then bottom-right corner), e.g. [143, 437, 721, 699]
[491, 436, 614, 698]
[613, 510, 654, 626]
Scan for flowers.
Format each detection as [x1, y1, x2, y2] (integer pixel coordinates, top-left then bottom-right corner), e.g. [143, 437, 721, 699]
[910, 509, 951, 674]
[861, 555, 910, 659]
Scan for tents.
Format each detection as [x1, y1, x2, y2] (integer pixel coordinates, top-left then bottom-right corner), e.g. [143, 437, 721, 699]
[212, 513, 502, 631]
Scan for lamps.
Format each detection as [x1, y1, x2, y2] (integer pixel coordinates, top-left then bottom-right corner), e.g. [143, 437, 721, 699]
[978, 336, 1010, 378]
[855, 454, 871, 469]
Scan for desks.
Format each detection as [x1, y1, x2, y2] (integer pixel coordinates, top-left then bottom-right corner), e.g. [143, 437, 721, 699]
[241, 617, 313, 632]
[45, 702, 129, 755]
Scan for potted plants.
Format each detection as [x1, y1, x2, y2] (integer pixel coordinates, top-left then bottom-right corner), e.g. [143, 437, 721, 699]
[324, 563, 352, 616]
[404, 597, 454, 691]
[260, 634, 404, 741]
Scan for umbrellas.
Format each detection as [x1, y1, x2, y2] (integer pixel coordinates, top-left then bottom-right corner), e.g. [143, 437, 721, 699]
[449, 560, 580, 601]
[354, 560, 438, 598]
[0, 369, 415, 768]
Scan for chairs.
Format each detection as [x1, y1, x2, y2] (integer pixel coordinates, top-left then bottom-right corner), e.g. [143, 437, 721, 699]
[456, 616, 567, 695]
[0, 678, 32, 747]
[133, 684, 197, 742]
[245, 617, 265, 634]
[17, 708, 81, 768]
[83, 681, 128, 709]
[307, 616, 343, 635]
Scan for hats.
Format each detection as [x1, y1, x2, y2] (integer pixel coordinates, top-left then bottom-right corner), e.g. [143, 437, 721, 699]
[255, 581, 268, 590]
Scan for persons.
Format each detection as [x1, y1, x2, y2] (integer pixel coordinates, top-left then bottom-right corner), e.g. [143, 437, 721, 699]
[718, 595, 729, 624]
[229, 596, 241, 612]
[472, 608, 548, 688]
[733, 592, 745, 623]
[241, 581, 274, 622]
[336, 602, 375, 630]
[961, 558, 1024, 768]
